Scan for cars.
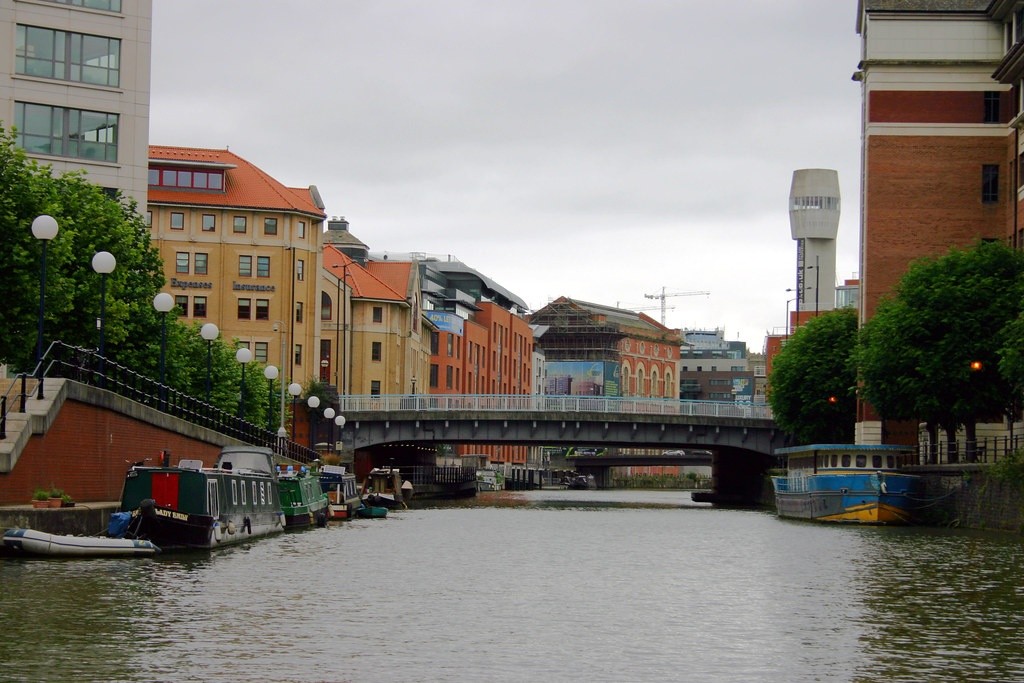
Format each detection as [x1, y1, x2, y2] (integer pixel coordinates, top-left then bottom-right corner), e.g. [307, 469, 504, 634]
[661, 448, 713, 455]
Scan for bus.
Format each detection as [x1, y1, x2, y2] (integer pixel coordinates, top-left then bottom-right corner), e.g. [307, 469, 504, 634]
[541, 446, 610, 467]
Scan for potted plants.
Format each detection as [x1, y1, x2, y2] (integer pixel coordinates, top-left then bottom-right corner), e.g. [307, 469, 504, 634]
[30, 483, 76, 508]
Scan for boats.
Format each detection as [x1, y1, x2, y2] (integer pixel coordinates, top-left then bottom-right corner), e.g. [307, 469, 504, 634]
[357, 505, 388, 519]
[119, 446, 289, 551]
[771, 445, 922, 526]
[358, 467, 410, 509]
[565, 474, 597, 490]
[314, 466, 363, 520]
[3, 528, 163, 558]
[475, 468, 504, 492]
[276, 464, 330, 528]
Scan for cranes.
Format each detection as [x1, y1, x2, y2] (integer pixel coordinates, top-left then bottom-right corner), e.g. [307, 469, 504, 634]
[645, 287, 712, 327]
[615, 301, 674, 311]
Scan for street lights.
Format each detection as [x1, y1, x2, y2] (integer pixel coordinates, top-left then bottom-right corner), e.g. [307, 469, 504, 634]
[153, 292, 175, 410]
[787, 287, 813, 327]
[286, 246, 295, 384]
[332, 260, 358, 410]
[201, 323, 219, 427]
[307, 395, 319, 464]
[325, 408, 334, 457]
[807, 265, 819, 318]
[236, 347, 252, 439]
[32, 215, 59, 376]
[335, 414, 345, 461]
[289, 382, 301, 455]
[92, 251, 116, 389]
[264, 365, 278, 444]
